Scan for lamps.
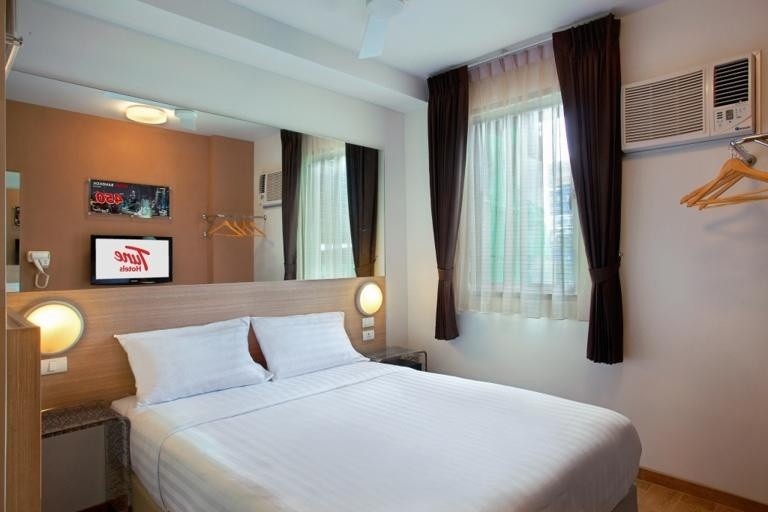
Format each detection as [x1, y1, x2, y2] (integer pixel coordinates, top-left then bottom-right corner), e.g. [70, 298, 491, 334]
[20, 297, 84, 358]
[123, 104, 168, 124]
[355, 280, 382, 315]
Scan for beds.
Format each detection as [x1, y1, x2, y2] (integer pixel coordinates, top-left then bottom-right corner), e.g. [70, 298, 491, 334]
[110, 306, 646, 512]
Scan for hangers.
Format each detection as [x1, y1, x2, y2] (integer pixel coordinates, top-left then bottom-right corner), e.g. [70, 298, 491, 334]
[200, 211, 268, 240]
[683, 137, 768, 211]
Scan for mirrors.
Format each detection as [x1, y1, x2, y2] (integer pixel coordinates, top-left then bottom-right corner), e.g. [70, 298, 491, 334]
[5, 168, 23, 292]
[3, 71, 385, 290]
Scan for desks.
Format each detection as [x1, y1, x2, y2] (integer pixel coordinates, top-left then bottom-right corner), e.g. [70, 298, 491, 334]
[361, 344, 427, 372]
[38, 402, 133, 512]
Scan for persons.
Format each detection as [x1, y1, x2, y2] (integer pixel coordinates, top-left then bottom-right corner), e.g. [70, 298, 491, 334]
[93, 187, 161, 219]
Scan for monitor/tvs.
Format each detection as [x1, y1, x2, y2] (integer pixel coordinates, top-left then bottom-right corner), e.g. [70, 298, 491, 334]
[90, 234, 173, 286]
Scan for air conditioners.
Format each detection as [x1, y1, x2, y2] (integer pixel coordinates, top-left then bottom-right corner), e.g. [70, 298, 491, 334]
[620, 51, 756, 154]
[258, 170, 282, 209]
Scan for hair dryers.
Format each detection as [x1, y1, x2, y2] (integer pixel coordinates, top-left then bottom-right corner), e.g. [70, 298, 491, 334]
[31, 252, 50, 273]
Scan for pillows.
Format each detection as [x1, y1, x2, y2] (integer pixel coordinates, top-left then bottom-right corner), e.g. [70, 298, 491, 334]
[113, 312, 274, 404]
[250, 309, 370, 378]
